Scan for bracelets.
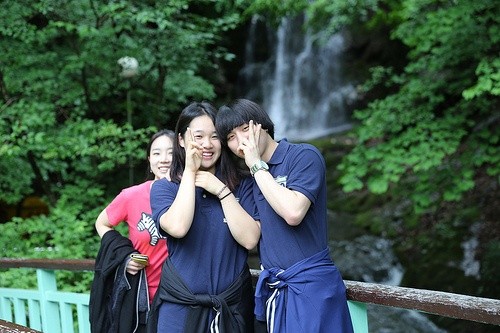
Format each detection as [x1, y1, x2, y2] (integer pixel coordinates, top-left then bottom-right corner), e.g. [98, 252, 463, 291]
[217, 184, 227, 197]
[219, 191, 233, 201]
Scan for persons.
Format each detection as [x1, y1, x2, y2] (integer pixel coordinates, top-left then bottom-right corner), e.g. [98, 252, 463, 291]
[95, 129, 175, 333]
[148, 101, 261, 333]
[215, 97, 354, 333]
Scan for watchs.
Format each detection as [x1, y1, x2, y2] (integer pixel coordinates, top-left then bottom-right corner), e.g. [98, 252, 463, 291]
[250, 161, 269, 177]
